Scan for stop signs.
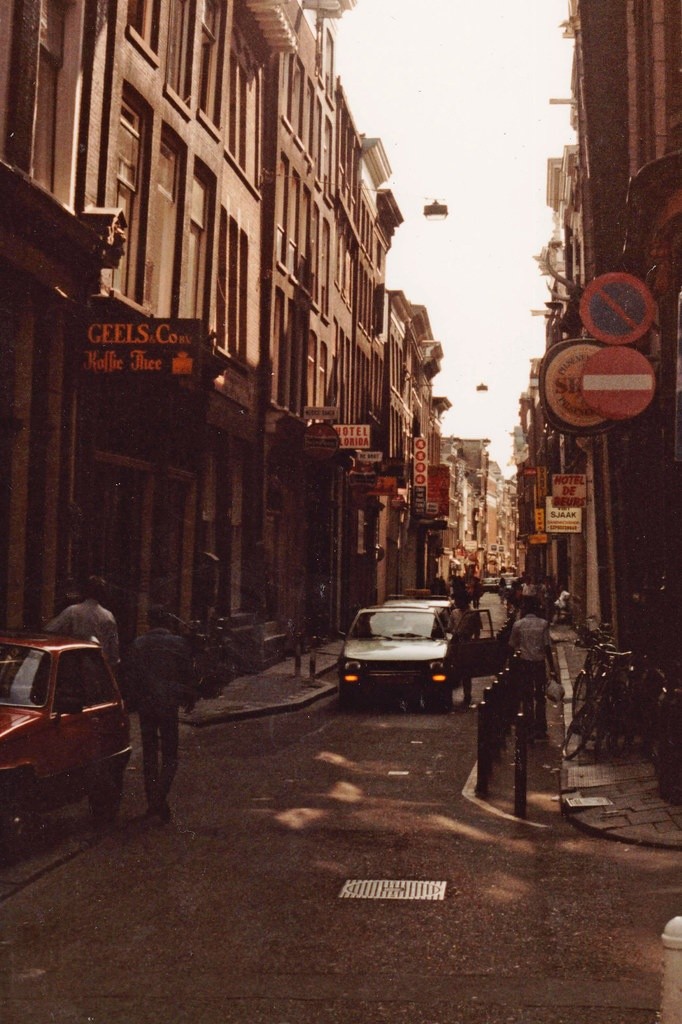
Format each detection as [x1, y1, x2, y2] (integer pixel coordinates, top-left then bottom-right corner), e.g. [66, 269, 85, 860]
[580, 342, 654, 420]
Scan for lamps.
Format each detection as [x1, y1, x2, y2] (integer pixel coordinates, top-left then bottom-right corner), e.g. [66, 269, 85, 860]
[423, 201, 448, 221]
[477, 384, 489, 393]
[80, 207, 128, 236]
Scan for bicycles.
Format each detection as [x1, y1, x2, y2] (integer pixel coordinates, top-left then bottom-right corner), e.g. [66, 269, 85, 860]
[561, 621, 682, 803]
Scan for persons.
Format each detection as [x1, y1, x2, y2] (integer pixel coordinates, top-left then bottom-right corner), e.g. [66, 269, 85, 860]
[124, 604, 197, 824]
[506, 571, 557, 621]
[443, 591, 483, 704]
[448, 575, 469, 600]
[43, 574, 121, 670]
[497, 577, 507, 604]
[471, 577, 481, 609]
[508, 595, 555, 744]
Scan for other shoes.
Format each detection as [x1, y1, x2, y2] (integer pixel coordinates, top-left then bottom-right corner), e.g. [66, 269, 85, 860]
[459, 695, 472, 712]
[159, 800, 169, 815]
[534, 731, 550, 740]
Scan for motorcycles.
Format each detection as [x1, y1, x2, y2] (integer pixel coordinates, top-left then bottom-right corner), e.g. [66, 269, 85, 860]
[169, 611, 232, 713]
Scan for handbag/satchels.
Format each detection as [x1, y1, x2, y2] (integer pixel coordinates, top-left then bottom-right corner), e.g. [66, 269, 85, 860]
[544, 675, 565, 702]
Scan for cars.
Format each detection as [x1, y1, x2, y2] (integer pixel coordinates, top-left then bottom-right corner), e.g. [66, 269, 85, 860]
[0, 629, 134, 861]
[337, 599, 495, 711]
[481, 575, 518, 592]
